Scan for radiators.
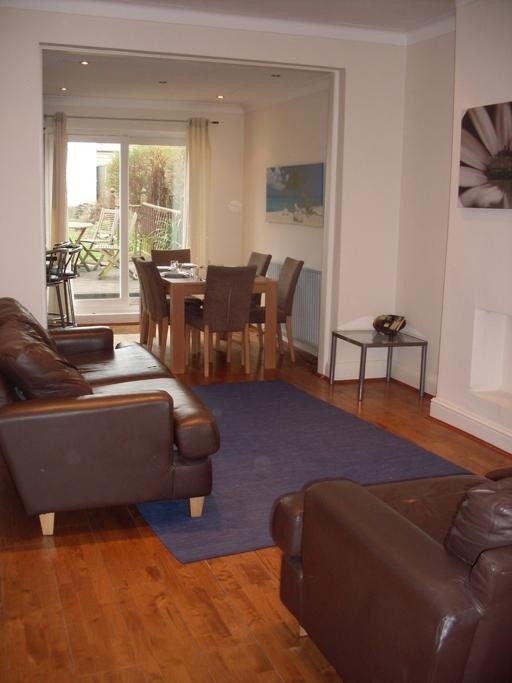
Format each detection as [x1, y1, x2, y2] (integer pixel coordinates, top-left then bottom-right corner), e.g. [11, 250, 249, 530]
[266, 259, 321, 357]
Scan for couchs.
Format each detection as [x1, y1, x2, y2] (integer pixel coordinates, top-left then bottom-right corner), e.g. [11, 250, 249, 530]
[269, 466, 511, 682]
[1, 296, 222, 535]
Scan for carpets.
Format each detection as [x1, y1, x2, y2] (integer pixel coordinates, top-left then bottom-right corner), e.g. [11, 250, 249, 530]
[133, 378, 477, 565]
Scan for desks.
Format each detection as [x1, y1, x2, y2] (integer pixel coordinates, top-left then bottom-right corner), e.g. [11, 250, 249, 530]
[68, 222, 103, 272]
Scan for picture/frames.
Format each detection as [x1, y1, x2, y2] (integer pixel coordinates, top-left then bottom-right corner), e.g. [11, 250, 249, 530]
[264, 161, 325, 230]
[455, 100, 511, 212]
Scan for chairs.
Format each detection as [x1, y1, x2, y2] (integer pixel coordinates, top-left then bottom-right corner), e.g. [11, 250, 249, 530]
[94, 211, 140, 281]
[137, 259, 215, 366]
[75, 208, 120, 271]
[150, 248, 190, 267]
[226, 256, 305, 375]
[185, 264, 259, 378]
[209, 249, 273, 352]
[46, 241, 83, 328]
[132, 256, 148, 344]
